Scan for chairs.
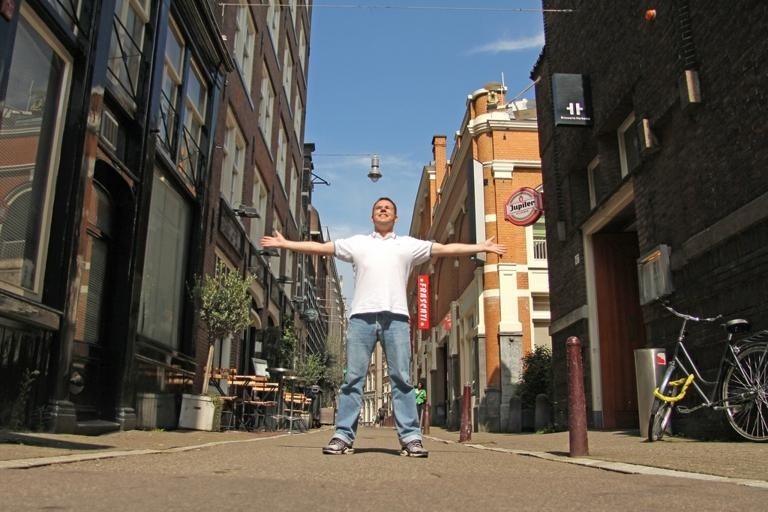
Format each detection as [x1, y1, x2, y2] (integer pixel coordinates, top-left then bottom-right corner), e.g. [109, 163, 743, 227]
[209, 365, 312, 433]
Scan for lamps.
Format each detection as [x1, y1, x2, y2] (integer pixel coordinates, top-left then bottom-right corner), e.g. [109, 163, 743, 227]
[306, 152, 382, 187]
[232, 202, 261, 220]
[310, 278, 342, 325]
[276, 275, 295, 285]
[289, 293, 304, 305]
[257, 247, 280, 257]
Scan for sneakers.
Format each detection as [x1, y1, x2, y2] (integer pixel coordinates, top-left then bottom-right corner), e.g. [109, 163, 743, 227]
[321, 438, 356, 455]
[400, 440, 428, 457]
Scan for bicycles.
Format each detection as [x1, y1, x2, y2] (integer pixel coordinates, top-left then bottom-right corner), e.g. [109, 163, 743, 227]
[645, 295, 767, 443]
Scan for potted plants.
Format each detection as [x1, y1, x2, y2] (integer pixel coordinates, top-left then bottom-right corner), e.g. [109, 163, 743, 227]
[317, 364, 346, 425]
[173, 260, 256, 433]
[291, 350, 325, 431]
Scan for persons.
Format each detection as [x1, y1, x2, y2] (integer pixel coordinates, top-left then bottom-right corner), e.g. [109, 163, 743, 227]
[413, 382, 427, 419]
[261, 197, 508, 458]
[379, 405, 388, 427]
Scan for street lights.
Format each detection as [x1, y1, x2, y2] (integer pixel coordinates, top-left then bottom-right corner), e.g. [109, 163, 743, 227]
[312, 152, 382, 183]
